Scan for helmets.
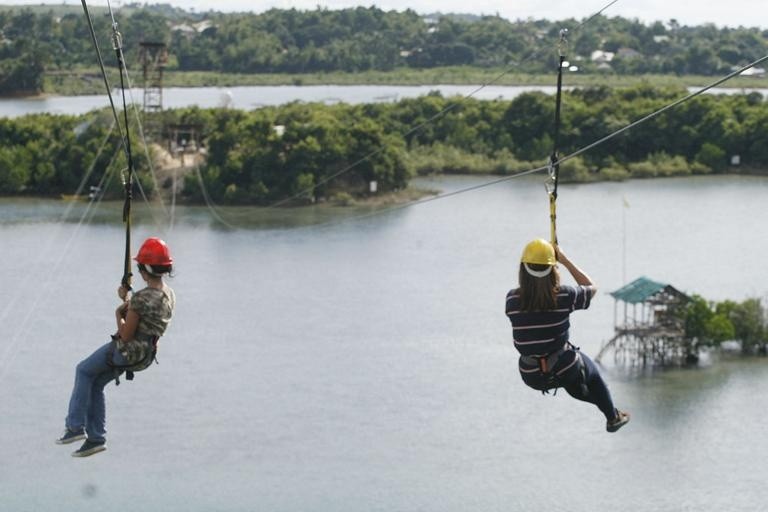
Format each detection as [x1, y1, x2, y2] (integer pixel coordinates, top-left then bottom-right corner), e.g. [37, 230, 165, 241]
[133, 238, 172, 266]
[522, 241, 556, 265]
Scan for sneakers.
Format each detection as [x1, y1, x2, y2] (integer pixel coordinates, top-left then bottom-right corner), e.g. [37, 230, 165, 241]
[607, 407, 629, 431]
[56, 427, 87, 444]
[72, 438, 106, 457]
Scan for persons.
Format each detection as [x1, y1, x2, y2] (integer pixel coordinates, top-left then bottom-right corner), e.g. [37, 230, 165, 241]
[53, 235, 178, 459]
[504, 239, 634, 432]
